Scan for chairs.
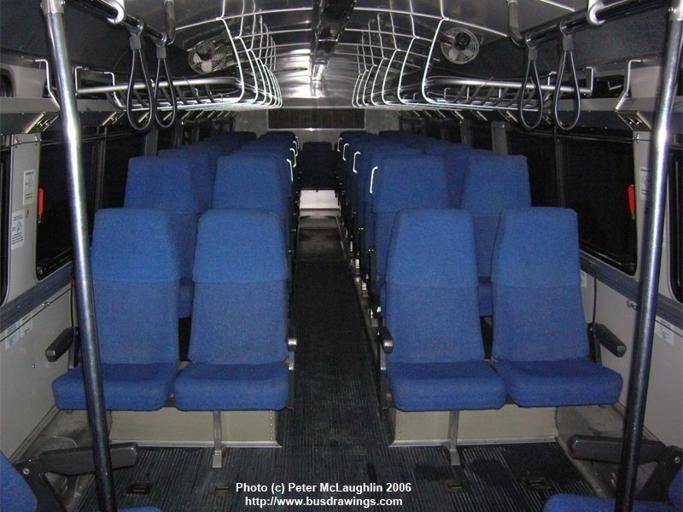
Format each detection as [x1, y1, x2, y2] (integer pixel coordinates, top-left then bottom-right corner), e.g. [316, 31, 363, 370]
[540, 444, 682, 511]
[0, 447, 163, 511]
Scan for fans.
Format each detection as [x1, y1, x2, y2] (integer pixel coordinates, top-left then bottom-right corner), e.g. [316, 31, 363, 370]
[185, 37, 229, 74]
[441, 26, 481, 65]
